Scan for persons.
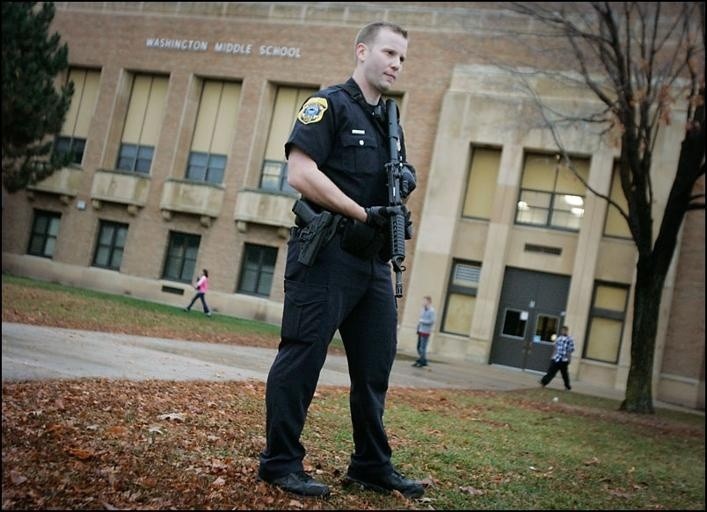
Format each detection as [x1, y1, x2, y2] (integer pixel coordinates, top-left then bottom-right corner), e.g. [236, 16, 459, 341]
[184, 269, 212, 318]
[410, 296, 436, 367]
[252, 20, 430, 500]
[535, 326, 574, 390]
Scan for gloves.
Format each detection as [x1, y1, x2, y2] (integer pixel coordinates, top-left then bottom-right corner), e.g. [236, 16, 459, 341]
[396, 162, 416, 199]
[363, 205, 413, 240]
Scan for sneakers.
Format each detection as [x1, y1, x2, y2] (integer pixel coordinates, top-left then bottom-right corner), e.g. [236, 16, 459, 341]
[413, 362, 423, 367]
[256, 471, 329, 500]
[344, 470, 425, 498]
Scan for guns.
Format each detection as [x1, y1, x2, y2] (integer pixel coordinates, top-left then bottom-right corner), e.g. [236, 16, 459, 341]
[290, 198, 332, 268]
[386, 98, 405, 298]
